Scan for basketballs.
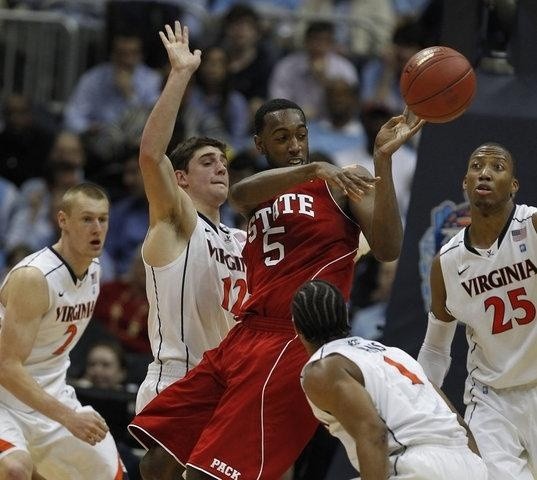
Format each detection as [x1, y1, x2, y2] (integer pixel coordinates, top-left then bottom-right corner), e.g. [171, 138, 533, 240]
[400, 46, 476, 124]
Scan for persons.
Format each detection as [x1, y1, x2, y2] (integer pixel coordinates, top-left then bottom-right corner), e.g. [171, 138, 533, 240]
[6, 158, 115, 286]
[0, 182, 128, 480]
[134, 19, 250, 417]
[219, 1, 296, 102]
[291, 278, 489, 480]
[268, 18, 361, 130]
[105, 144, 152, 275]
[126, 98, 425, 480]
[417, 141, 535, 480]
[77, 342, 133, 440]
[53, 29, 162, 178]
[0, 93, 60, 188]
[176, 42, 254, 228]
